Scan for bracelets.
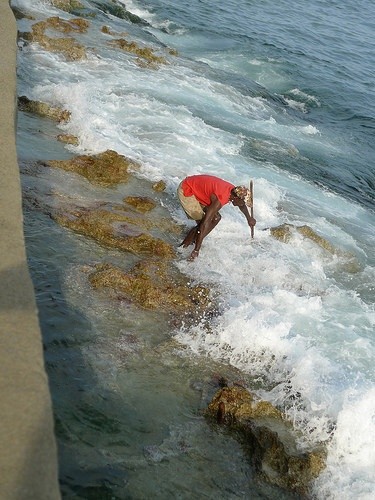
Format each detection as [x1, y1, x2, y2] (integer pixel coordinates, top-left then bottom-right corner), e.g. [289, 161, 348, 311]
[193, 250, 199, 253]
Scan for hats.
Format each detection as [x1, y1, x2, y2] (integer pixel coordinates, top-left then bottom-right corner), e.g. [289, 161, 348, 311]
[232, 185, 254, 208]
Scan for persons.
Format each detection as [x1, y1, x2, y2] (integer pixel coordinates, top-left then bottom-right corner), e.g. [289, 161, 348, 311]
[176, 174, 256, 261]
